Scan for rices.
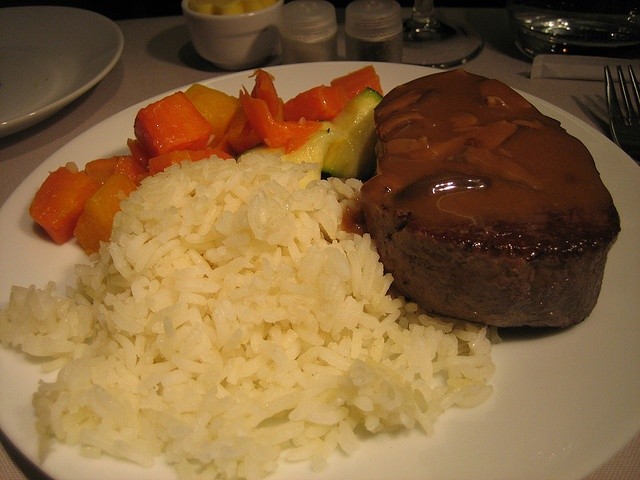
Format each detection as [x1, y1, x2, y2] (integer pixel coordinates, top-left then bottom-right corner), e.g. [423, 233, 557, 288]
[2, 152, 504, 480]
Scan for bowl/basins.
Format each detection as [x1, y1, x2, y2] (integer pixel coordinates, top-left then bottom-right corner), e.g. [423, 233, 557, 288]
[511, 9, 640, 61]
[178, 0, 285, 70]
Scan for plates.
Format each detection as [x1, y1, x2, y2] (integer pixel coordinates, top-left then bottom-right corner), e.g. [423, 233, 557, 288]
[0, 61, 638, 478]
[1, 2, 126, 132]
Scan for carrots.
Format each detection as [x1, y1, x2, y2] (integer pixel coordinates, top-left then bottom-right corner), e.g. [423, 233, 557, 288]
[36, 62, 385, 256]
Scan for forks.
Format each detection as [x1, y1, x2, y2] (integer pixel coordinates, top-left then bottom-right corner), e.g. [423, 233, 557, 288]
[602, 62, 639, 160]
